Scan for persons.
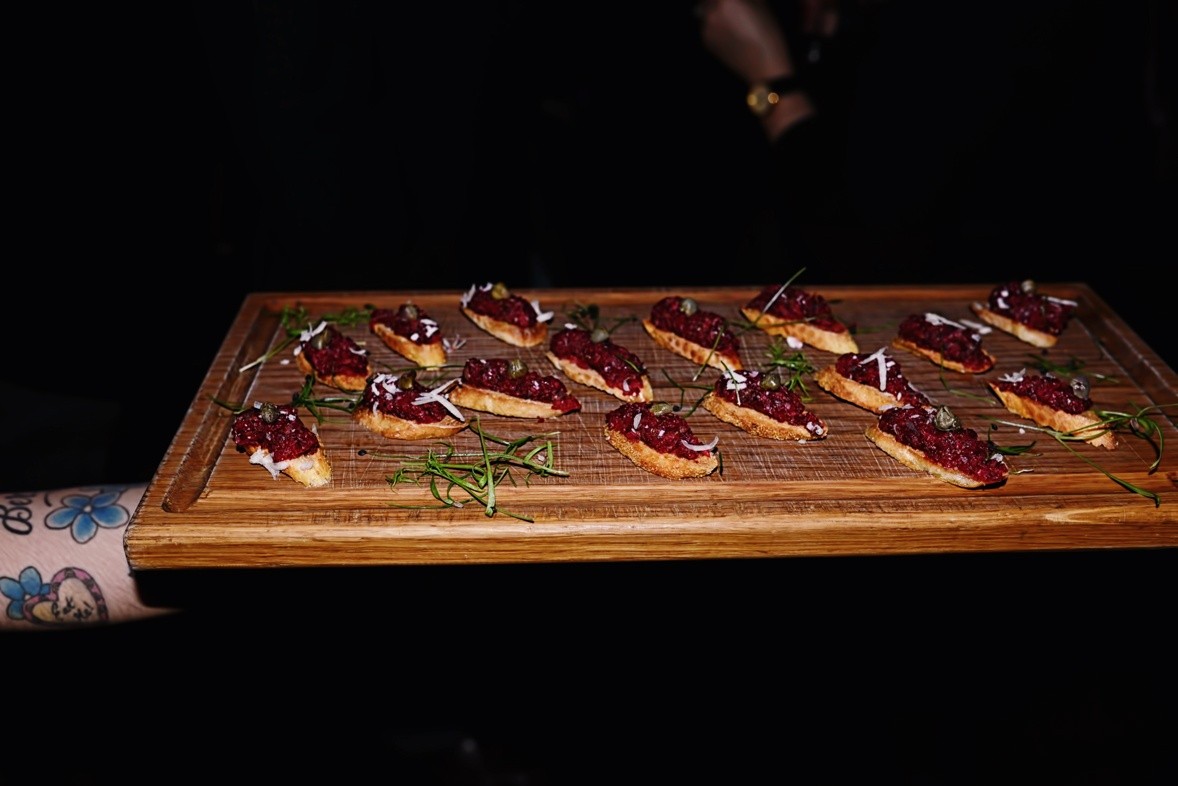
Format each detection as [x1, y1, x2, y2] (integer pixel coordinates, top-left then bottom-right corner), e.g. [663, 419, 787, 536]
[693, 0, 840, 145]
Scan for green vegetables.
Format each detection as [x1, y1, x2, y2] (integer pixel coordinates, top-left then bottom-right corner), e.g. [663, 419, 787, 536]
[214, 305, 1165, 503]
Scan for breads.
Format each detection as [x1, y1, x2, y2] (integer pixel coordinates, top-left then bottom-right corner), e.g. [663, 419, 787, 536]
[250, 293, 1117, 486]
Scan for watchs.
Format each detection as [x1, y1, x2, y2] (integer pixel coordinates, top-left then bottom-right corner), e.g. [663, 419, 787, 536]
[747, 73, 803, 116]
